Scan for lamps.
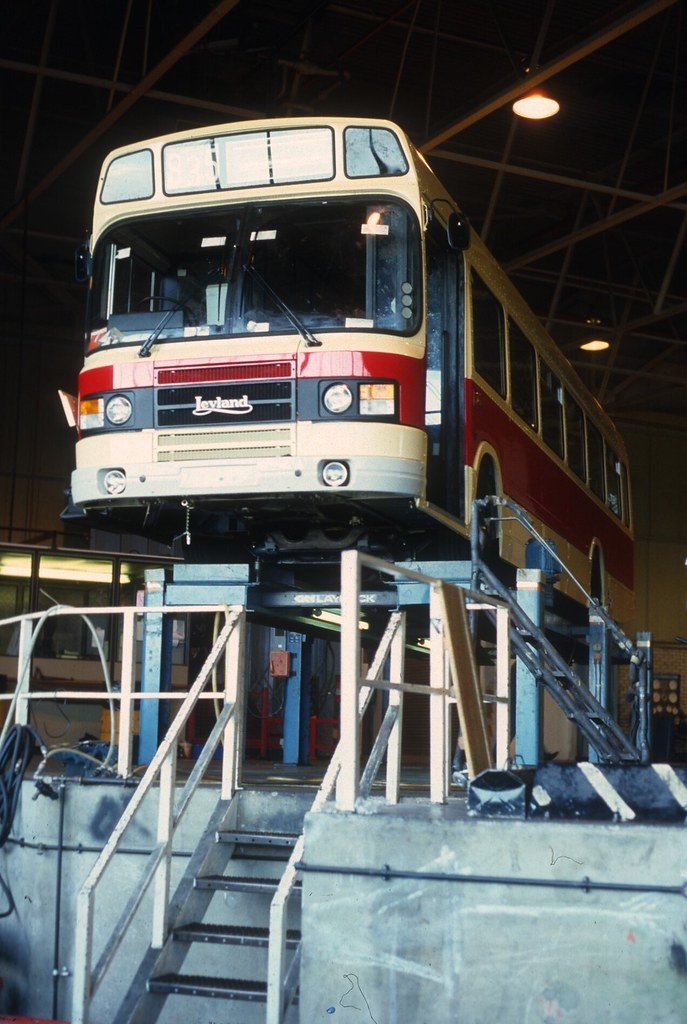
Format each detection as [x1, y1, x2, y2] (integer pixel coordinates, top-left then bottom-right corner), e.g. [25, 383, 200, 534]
[417, 638, 430, 649]
[513, 64, 560, 120]
[312, 607, 368, 629]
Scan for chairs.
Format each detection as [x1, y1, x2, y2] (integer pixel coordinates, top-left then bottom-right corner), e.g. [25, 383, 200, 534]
[160, 269, 207, 326]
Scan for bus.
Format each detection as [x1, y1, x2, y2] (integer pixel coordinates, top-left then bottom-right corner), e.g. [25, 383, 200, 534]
[56, 114, 654, 669]
